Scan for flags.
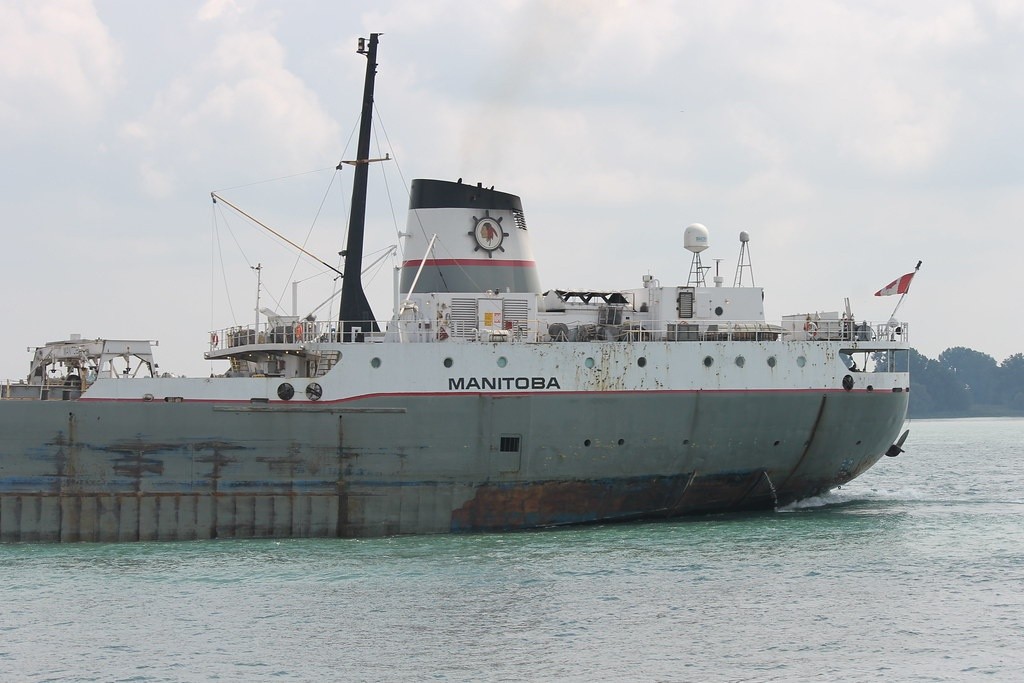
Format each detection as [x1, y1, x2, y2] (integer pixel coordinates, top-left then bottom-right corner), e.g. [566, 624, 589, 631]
[873, 272, 914, 296]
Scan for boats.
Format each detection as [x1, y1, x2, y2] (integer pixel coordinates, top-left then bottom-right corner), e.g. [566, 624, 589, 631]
[0, 33, 922, 542]
